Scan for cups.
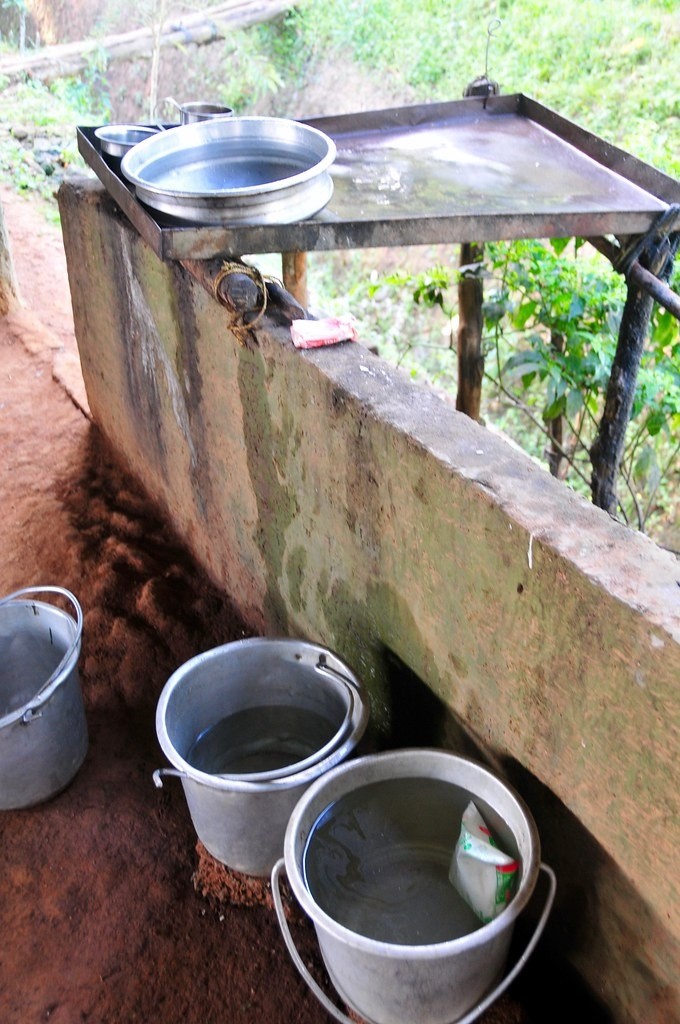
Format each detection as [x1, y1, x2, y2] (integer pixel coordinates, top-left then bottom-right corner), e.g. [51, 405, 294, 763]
[95, 125, 160, 193]
[153, 97, 233, 131]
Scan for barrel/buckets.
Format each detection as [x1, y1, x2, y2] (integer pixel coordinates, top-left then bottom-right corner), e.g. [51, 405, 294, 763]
[0, 586, 89, 811]
[152, 635, 370, 877]
[271, 749, 556, 1024]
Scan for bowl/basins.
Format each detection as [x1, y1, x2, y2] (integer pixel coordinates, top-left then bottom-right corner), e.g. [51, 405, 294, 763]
[120, 116, 337, 225]
